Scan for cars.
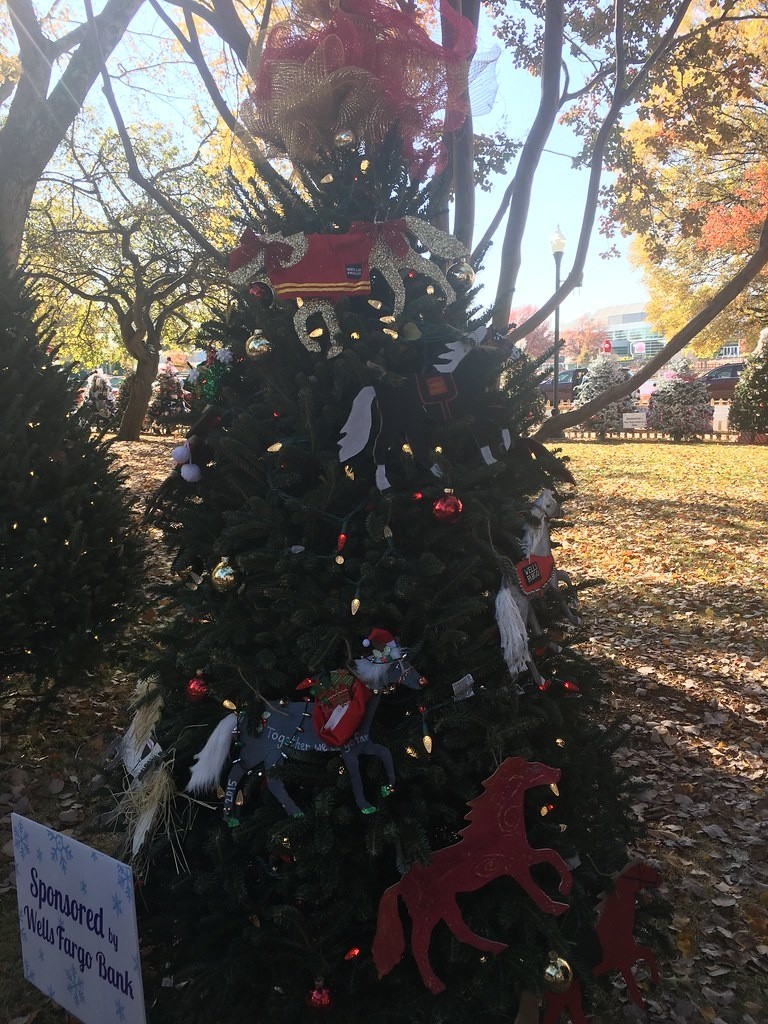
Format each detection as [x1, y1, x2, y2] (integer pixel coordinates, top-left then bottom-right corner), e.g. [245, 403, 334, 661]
[76, 373, 126, 408]
[689, 362, 747, 401]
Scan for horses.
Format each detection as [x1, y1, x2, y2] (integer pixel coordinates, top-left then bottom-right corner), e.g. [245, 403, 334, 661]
[490, 493, 572, 687]
[184, 648, 425, 825]
[223, 223, 466, 361]
[371, 760, 568, 995]
[516, 855, 670, 1024]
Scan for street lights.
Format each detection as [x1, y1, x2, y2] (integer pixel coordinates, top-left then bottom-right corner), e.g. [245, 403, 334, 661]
[550, 225, 566, 439]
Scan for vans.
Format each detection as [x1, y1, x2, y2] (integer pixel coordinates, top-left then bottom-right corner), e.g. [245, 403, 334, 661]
[540, 368, 635, 405]
